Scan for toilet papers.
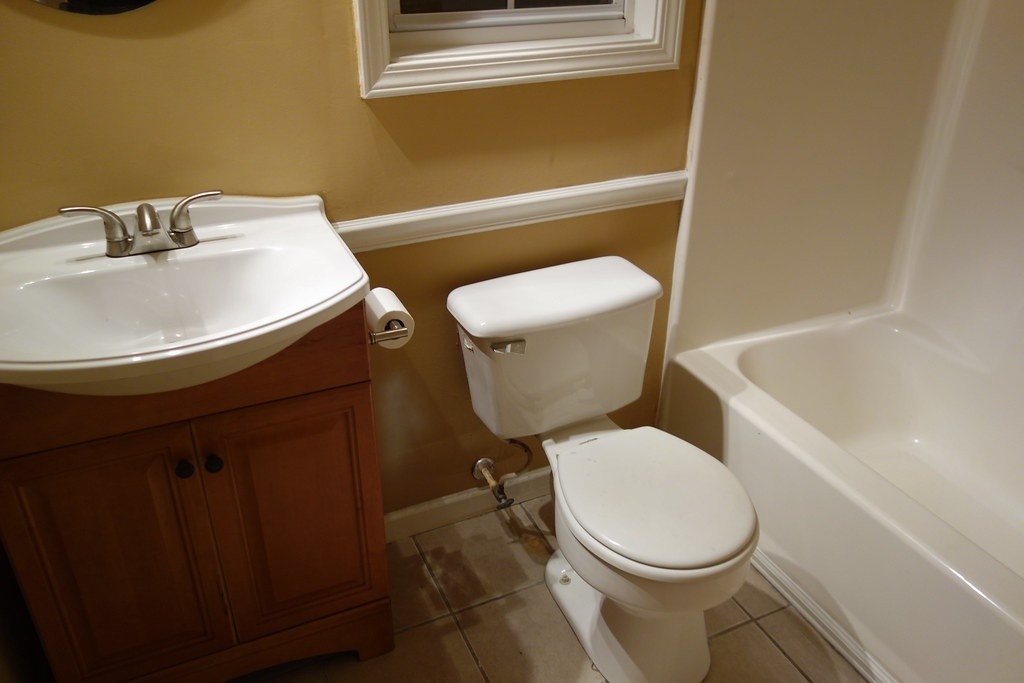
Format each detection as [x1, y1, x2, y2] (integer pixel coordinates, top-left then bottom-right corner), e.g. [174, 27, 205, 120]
[364, 286, 415, 351]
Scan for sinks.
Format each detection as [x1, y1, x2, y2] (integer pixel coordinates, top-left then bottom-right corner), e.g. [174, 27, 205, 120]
[1, 195, 370, 396]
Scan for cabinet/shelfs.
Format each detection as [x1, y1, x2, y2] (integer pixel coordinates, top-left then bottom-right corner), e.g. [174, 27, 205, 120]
[0, 297, 395, 683]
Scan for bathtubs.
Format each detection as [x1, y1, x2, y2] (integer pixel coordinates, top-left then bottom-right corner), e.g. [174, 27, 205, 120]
[668, 307, 1020, 682]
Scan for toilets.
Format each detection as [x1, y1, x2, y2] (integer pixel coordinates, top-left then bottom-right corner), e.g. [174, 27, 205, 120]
[442, 254, 761, 683]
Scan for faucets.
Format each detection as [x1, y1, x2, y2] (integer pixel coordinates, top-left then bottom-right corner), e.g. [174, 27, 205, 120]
[127, 202, 178, 255]
